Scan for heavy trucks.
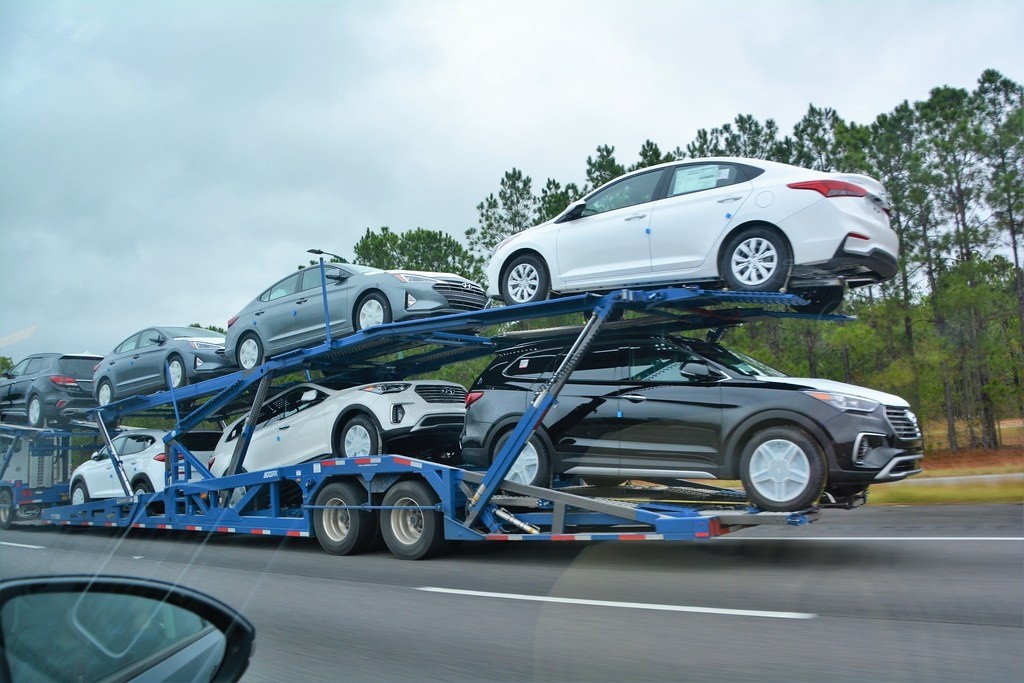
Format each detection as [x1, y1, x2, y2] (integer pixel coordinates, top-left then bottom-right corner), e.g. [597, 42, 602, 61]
[1, 284, 822, 540]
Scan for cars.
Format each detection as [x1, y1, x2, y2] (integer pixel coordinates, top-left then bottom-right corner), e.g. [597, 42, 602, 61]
[1, 351, 104, 427]
[211, 380, 470, 468]
[70, 430, 224, 508]
[226, 263, 485, 371]
[93, 326, 234, 407]
[463, 343, 926, 512]
[483, 156, 901, 315]
